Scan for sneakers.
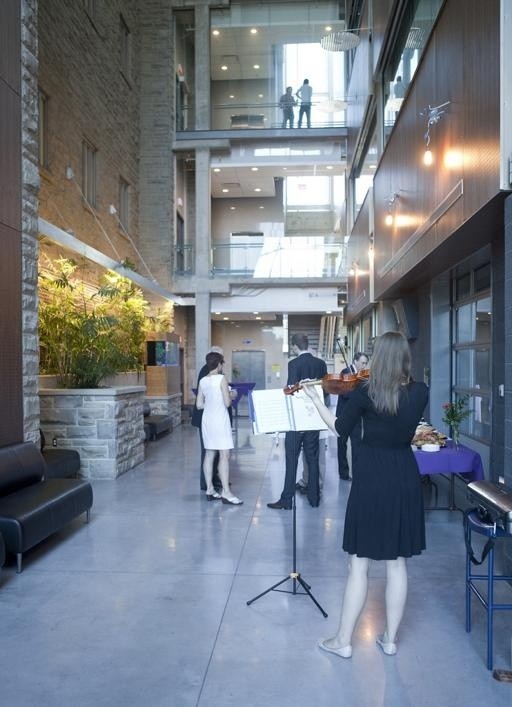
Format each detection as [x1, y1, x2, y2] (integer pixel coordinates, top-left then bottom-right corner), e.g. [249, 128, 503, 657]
[296, 482, 319, 507]
[200, 478, 232, 493]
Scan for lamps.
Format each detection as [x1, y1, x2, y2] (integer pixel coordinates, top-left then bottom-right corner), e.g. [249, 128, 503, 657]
[383, 189, 402, 225]
[320, 27, 369, 52]
[415, 99, 451, 165]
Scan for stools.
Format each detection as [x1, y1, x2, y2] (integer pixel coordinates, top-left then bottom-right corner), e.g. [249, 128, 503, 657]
[465, 510, 512, 670]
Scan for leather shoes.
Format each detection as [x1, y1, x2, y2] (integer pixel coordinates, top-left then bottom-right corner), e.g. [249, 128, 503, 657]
[266, 499, 293, 511]
[315, 638, 354, 658]
[374, 632, 399, 657]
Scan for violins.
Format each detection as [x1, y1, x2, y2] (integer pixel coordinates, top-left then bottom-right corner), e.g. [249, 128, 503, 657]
[284, 368, 371, 395]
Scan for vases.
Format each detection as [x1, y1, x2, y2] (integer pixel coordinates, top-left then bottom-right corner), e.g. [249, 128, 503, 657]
[451, 420, 460, 452]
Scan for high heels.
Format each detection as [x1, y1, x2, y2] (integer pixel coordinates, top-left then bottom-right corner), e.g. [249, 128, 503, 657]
[206, 490, 221, 503]
[221, 494, 244, 507]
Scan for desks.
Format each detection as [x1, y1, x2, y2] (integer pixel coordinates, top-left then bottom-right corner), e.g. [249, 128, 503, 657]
[192, 382, 256, 417]
[411, 422, 485, 512]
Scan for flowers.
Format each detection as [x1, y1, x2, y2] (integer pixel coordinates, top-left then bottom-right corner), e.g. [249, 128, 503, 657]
[441, 393, 474, 440]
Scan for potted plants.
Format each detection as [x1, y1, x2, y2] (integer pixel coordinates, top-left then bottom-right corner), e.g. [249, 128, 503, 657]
[38, 230, 176, 480]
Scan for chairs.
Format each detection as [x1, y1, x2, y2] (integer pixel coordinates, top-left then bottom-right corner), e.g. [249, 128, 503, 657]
[144, 405, 172, 440]
[39, 428, 80, 480]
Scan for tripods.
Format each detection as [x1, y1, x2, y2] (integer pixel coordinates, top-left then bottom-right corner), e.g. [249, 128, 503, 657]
[247, 432, 328, 618]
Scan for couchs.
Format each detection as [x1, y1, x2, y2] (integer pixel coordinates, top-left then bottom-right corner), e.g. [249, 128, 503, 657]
[0, 441, 93, 574]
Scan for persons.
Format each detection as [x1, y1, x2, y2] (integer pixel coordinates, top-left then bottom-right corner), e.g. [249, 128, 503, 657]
[392, 75, 405, 98]
[279, 86, 298, 128]
[295, 78, 312, 128]
[191, 346, 231, 492]
[335, 352, 369, 481]
[266, 331, 328, 511]
[295, 393, 331, 494]
[195, 351, 245, 505]
[317, 330, 430, 660]
